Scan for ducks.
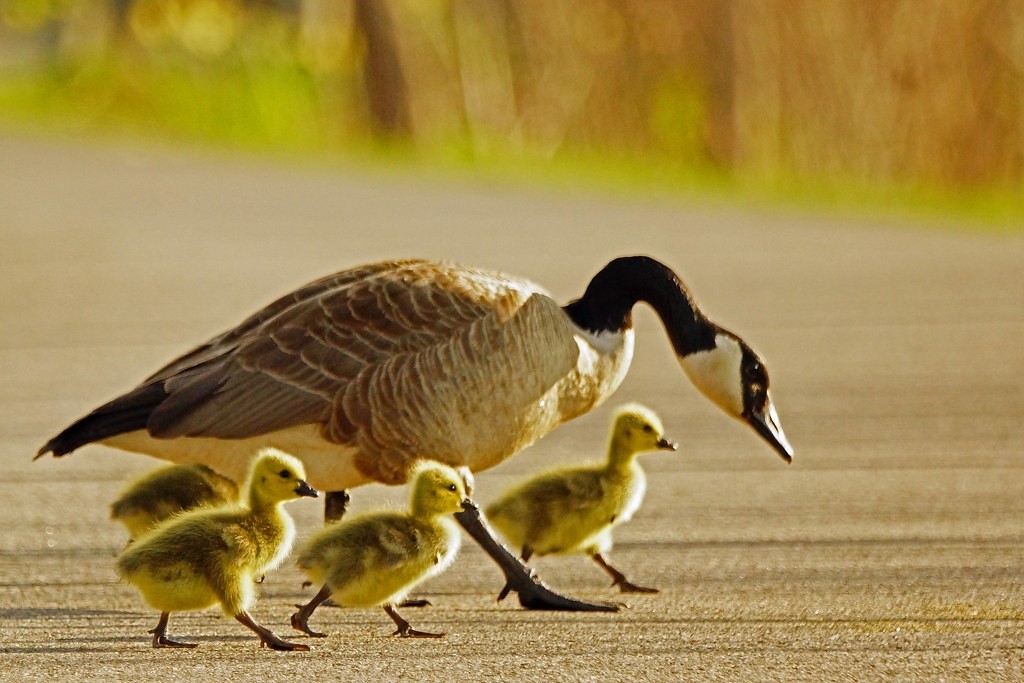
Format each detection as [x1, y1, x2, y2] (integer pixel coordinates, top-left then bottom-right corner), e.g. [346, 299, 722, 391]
[32, 255, 794, 653]
